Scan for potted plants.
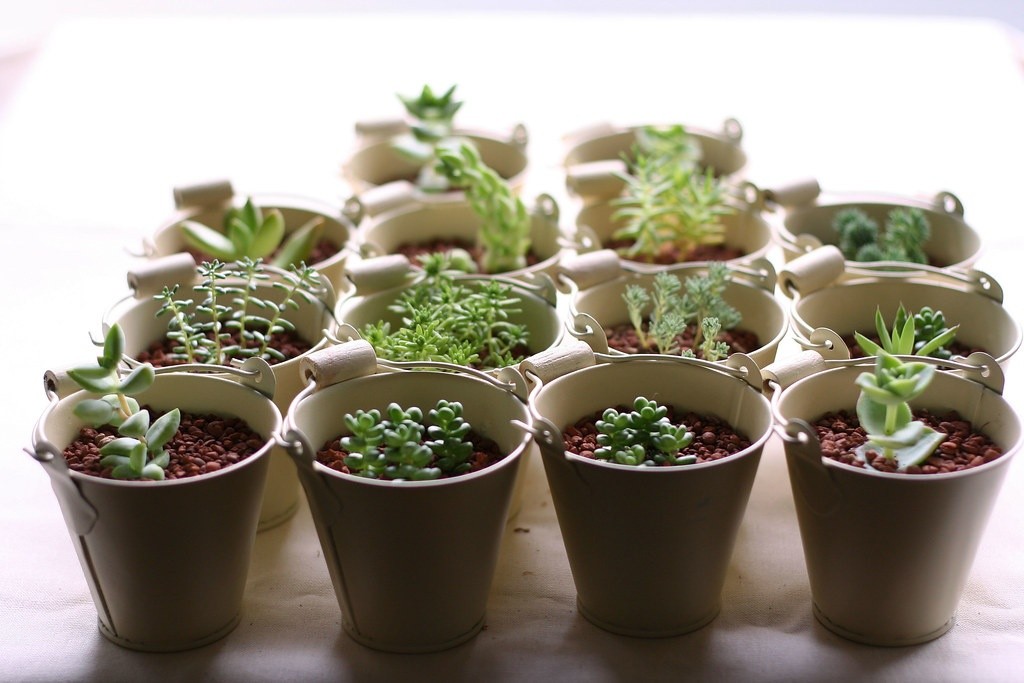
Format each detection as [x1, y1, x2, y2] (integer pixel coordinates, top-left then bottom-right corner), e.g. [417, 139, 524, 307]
[23, 84, 1024, 655]
